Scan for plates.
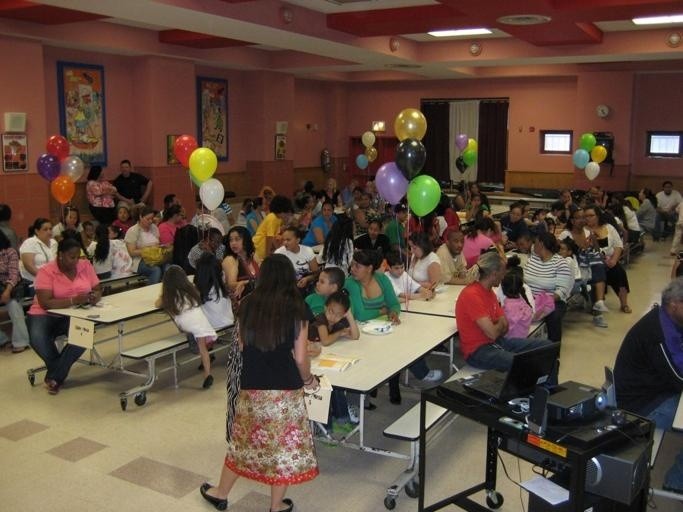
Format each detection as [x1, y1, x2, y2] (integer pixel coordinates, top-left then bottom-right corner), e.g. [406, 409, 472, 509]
[362, 324, 394, 335]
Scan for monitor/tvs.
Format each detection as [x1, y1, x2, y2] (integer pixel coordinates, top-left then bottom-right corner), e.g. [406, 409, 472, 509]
[649, 134, 681, 155]
[543, 133, 571, 152]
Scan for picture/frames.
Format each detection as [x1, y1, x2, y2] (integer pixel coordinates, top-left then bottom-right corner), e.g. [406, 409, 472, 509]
[56, 59, 107, 168]
[195, 75, 229, 160]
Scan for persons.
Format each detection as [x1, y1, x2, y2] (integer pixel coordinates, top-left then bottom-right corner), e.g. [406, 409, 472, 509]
[612, 280, 683, 497]
[112, 158, 153, 214]
[1, 174, 683, 439]
[85, 164, 118, 227]
[197, 252, 318, 511]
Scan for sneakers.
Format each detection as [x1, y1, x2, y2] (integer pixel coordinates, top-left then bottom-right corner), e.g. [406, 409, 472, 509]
[423, 370, 442, 382]
[593, 300, 608, 311]
[593, 315, 608, 327]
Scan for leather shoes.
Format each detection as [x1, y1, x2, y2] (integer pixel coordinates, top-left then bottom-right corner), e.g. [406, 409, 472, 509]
[45, 374, 60, 395]
[200, 484, 227, 510]
[270, 499, 292, 512]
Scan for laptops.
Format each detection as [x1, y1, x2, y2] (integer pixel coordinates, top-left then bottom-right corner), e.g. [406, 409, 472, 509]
[464, 343, 561, 401]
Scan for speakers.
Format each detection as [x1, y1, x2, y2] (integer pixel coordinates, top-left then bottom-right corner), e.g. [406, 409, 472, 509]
[527, 387, 549, 438]
[586, 438, 652, 505]
[596, 366, 618, 412]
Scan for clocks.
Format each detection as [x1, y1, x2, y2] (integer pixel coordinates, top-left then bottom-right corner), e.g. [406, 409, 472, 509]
[595, 104, 609, 118]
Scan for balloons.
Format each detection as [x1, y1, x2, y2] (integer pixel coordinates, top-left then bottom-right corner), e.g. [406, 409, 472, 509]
[44, 134, 68, 161]
[172, 134, 196, 169]
[188, 147, 218, 183]
[36, 154, 58, 181]
[454, 134, 478, 173]
[355, 104, 445, 219]
[59, 155, 84, 183]
[572, 131, 609, 182]
[188, 170, 201, 189]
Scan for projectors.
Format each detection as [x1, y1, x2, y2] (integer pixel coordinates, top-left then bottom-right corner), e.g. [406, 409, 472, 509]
[531, 380, 607, 425]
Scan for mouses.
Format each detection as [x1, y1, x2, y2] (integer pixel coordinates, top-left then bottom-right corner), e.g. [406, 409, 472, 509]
[611, 409, 627, 425]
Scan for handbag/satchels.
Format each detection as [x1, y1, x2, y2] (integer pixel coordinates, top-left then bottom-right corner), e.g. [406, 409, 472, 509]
[534, 292, 555, 322]
[141, 246, 164, 265]
[15, 278, 33, 301]
